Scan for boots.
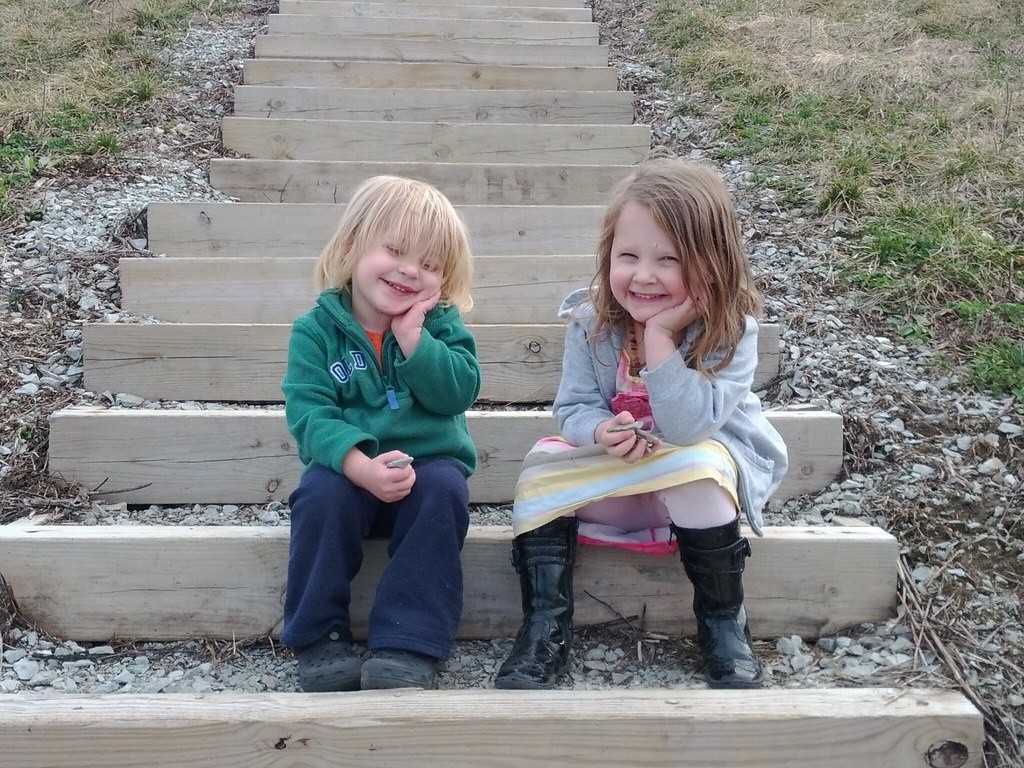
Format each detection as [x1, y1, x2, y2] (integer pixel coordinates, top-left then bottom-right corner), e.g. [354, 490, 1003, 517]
[669, 512, 763, 688]
[495, 515, 580, 688]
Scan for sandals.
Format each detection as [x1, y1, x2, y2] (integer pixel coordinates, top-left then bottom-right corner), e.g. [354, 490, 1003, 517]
[360, 648, 438, 690]
[294, 626, 361, 691]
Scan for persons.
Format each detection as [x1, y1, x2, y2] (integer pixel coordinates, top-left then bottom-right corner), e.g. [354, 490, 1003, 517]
[493, 163, 788, 690]
[280, 176, 480, 691]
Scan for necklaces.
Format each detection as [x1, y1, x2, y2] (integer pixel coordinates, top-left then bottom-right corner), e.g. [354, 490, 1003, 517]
[628, 316, 641, 372]
